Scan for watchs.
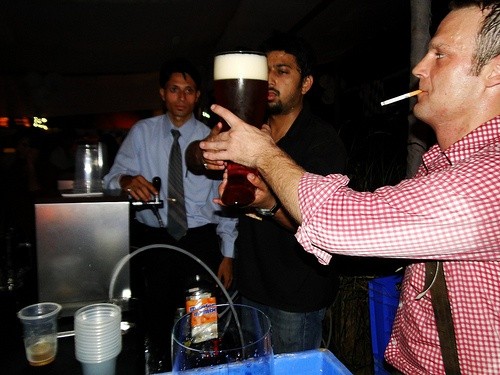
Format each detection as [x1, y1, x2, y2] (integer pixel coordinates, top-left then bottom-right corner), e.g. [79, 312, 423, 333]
[256, 201, 281, 216]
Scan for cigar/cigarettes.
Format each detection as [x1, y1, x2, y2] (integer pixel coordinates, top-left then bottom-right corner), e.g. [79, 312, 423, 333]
[381, 89, 423, 106]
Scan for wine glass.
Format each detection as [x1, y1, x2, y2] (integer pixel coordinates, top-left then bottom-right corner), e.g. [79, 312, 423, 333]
[212, 49, 269, 216]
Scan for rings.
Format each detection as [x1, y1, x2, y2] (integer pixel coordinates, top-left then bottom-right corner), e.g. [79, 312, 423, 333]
[204, 162, 208, 169]
[128, 189, 132, 192]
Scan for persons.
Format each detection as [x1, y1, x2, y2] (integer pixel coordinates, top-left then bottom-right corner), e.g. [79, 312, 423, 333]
[185, 32, 347, 358]
[103, 57, 239, 375]
[199, 0, 500, 375]
[0, 97, 168, 197]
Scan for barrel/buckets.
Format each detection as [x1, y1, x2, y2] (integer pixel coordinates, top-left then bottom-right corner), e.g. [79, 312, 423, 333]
[73, 143, 103, 193]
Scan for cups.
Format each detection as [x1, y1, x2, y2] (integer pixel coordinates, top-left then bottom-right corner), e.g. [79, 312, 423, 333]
[172, 303, 274, 375]
[17, 302, 62, 366]
[75, 303, 122, 375]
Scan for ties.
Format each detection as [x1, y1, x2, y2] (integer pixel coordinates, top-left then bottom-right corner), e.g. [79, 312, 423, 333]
[165, 129, 190, 240]
